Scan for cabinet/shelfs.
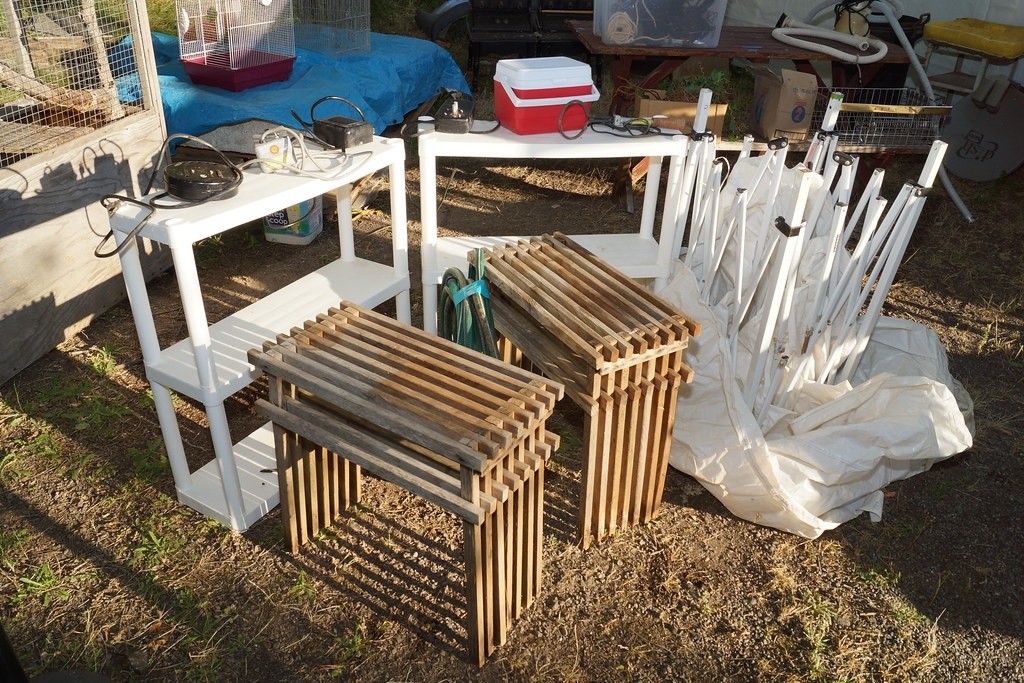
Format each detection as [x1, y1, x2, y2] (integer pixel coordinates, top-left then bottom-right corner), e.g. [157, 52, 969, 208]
[417, 114, 689, 338]
[107, 136, 412, 532]
[924, 20, 1024, 123]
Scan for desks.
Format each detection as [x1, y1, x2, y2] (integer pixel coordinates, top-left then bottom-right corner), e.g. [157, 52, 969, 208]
[566, 21, 928, 121]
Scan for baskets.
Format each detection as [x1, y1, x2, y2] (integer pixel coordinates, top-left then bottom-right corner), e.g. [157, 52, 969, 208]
[809, 87, 947, 151]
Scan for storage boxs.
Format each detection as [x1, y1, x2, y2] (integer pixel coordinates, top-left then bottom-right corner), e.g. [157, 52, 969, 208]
[634, 89, 729, 140]
[494, 55, 601, 134]
[594, 0, 727, 48]
[744, 58, 818, 141]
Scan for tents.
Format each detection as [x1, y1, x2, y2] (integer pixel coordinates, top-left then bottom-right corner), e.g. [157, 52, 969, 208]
[637, 86, 977, 540]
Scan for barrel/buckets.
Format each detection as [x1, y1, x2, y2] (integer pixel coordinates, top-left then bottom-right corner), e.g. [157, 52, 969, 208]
[264, 194, 326, 244]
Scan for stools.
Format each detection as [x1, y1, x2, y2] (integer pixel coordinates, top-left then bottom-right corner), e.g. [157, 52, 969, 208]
[469, 230, 699, 546]
[249, 299, 568, 666]
